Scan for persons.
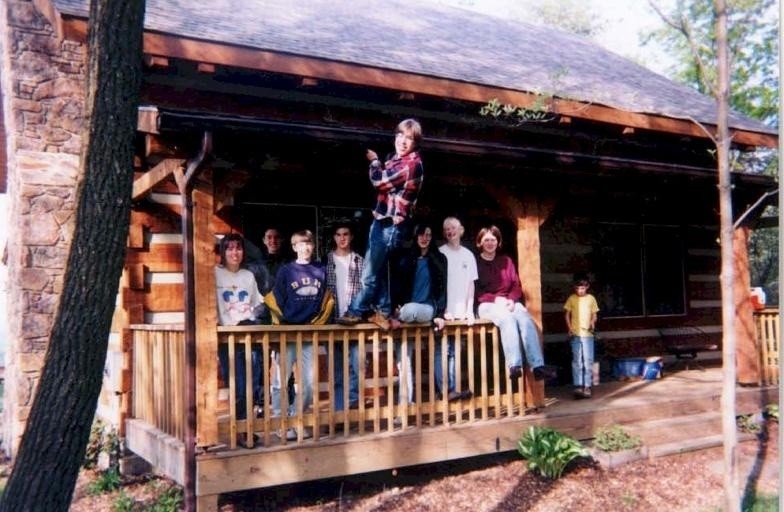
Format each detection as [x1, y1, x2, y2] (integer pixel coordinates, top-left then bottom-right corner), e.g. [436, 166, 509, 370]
[320, 221, 364, 434]
[428, 215, 481, 402]
[251, 222, 294, 416]
[390, 222, 448, 428]
[264, 228, 336, 445]
[214, 232, 263, 451]
[332, 117, 426, 323]
[472, 224, 557, 383]
[562, 277, 601, 398]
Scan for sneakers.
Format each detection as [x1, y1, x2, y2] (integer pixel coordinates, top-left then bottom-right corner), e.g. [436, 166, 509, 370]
[295, 427, 315, 437]
[509, 366, 522, 380]
[531, 365, 559, 381]
[334, 312, 364, 326]
[350, 397, 373, 409]
[438, 390, 462, 402]
[326, 424, 356, 432]
[584, 387, 592, 398]
[456, 389, 471, 400]
[275, 427, 298, 440]
[367, 315, 392, 332]
[574, 388, 583, 397]
[236, 433, 263, 447]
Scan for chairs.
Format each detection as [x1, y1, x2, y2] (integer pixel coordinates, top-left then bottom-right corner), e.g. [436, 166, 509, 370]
[655, 323, 719, 373]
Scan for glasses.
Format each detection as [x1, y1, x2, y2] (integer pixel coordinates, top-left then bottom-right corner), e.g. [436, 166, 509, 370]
[416, 233, 434, 242]
